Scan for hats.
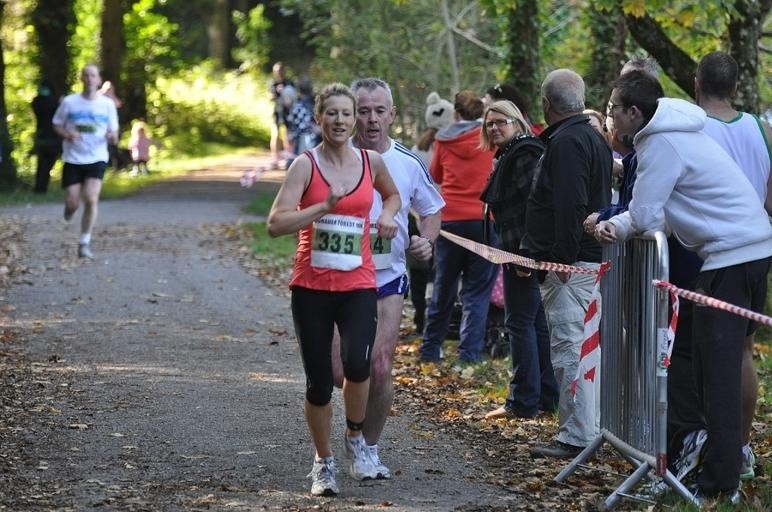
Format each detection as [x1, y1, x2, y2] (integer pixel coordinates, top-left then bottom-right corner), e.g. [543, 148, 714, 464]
[425, 91, 455, 130]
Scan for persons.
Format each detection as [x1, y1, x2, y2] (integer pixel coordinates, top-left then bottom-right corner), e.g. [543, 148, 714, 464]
[408, 52, 771, 510]
[127, 119, 161, 177]
[265, 81, 401, 495]
[329, 77, 447, 480]
[268, 62, 321, 171]
[48, 61, 121, 267]
[96, 79, 124, 171]
[28, 78, 62, 195]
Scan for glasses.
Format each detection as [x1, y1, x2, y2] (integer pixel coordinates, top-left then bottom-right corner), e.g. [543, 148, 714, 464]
[485, 118, 517, 128]
[493, 83, 502, 93]
[607, 100, 629, 111]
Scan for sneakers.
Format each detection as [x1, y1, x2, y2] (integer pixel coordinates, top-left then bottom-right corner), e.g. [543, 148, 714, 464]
[78, 243, 96, 258]
[310, 451, 340, 497]
[483, 401, 542, 420]
[528, 439, 601, 462]
[415, 321, 427, 335]
[438, 345, 446, 361]
[63, 207, 76, 221]
[367, 444, 391, 480]
[630, 429, 757, 507]
[343, 429, 378, 481]
